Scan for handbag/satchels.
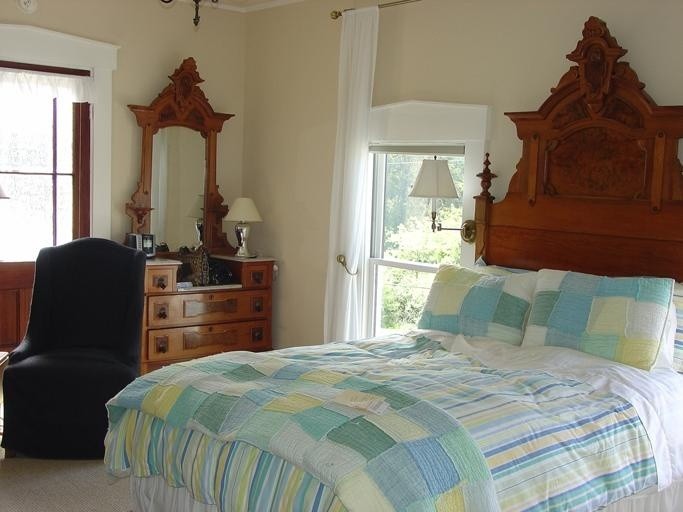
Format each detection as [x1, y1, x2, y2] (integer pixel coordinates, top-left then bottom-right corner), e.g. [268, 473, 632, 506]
[174, 249, 210, 286]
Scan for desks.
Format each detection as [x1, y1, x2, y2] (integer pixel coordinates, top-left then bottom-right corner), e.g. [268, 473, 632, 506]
[0, 350, 10, 370]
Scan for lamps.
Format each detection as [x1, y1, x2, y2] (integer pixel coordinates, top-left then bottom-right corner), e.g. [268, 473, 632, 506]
[405, 153, 476, 244]
[224, 194, 264, 260]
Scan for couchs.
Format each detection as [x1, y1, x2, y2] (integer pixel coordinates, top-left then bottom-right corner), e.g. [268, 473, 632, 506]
[0, 237, 145, 463]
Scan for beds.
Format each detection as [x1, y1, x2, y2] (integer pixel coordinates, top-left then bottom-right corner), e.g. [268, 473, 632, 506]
[97, 13, 682, 511]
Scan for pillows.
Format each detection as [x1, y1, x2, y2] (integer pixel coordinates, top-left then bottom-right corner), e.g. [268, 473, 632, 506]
[520, 267, 676, 372]
[419, 262, 538, 350]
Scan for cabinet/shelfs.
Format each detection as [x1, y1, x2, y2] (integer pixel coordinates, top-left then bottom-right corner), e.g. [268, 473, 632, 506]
[139, 252, 275, 377]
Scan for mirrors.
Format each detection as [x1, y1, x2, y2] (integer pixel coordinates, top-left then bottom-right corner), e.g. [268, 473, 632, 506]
[149, 124, 207, 253]
